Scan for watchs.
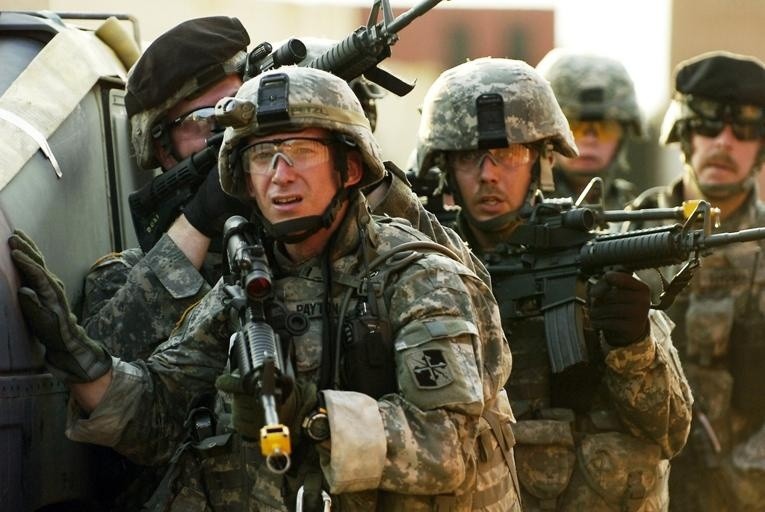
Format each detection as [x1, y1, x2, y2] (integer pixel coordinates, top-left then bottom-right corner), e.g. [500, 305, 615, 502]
[300, 398, 334, 448]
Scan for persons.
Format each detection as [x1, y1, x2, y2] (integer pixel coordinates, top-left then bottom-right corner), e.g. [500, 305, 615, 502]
[537, 44, 641, 235]
[619, 50, 765, 511]
[7, 64, 524, 511]
[410, 56, 695, 512]
[80, 15, 495, 365]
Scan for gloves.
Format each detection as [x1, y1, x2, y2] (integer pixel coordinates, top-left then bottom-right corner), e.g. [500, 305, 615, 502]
[8, 228, 112, 380]
[588, 271, 650, 344]
[215, 371, 317, 439]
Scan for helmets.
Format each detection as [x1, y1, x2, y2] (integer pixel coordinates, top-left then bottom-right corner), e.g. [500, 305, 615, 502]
[660, 52, 765, 145]
[218, 66, 385, 198]
[125, 16, 248, 169]
[535, 47, 643, 137]
[415, 57, 579, 178]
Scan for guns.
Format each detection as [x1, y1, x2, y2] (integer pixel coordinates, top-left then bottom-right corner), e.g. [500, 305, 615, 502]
[480, 200, 765, 381]
[222, 213, 310, 474]
[407, 168, 721, 243]
[128, 0, 440, 256]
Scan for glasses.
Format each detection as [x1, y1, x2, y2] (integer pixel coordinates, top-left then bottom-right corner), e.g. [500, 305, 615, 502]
[689, 118, 762, 140]
[163, 105, 218, 134]
[447, 143, 533, 171]
[238, 139, 334, 173]
